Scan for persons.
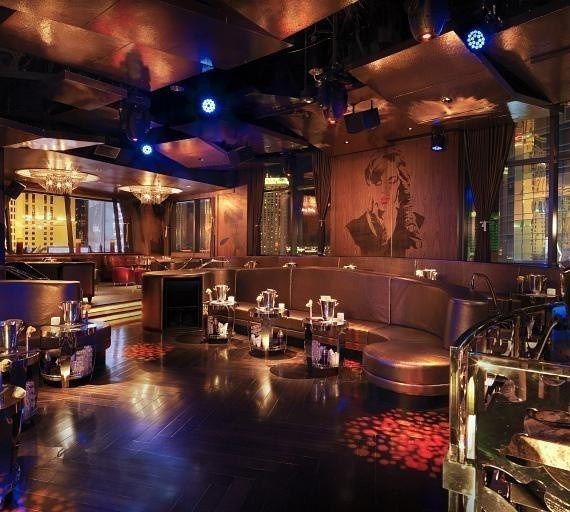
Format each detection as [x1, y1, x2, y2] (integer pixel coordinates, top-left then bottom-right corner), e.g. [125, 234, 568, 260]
[342, 146, 428, 258]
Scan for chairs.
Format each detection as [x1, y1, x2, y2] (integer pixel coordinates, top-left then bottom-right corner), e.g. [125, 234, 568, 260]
[57, 263, 95, 304]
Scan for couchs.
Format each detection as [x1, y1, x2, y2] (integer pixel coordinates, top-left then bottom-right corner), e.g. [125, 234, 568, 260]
[0, 280, 81, 347]
[202, 256, 565, 397]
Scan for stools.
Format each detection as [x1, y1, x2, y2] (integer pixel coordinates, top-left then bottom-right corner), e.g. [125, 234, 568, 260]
[134, 268, 150, 289]
[111, 267, 137, 288]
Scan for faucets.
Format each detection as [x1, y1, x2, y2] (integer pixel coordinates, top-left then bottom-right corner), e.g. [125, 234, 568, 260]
[471, 272, 499, 310]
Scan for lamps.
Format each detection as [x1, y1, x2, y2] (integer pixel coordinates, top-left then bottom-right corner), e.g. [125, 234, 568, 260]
[408, 0, 449, 43]
[117, 173, 184, 206]
[430, 125, 446, 152]
[344, 103, 364, 134]
[359, 99, 380, 129]
[14, 168, 101, 196]
